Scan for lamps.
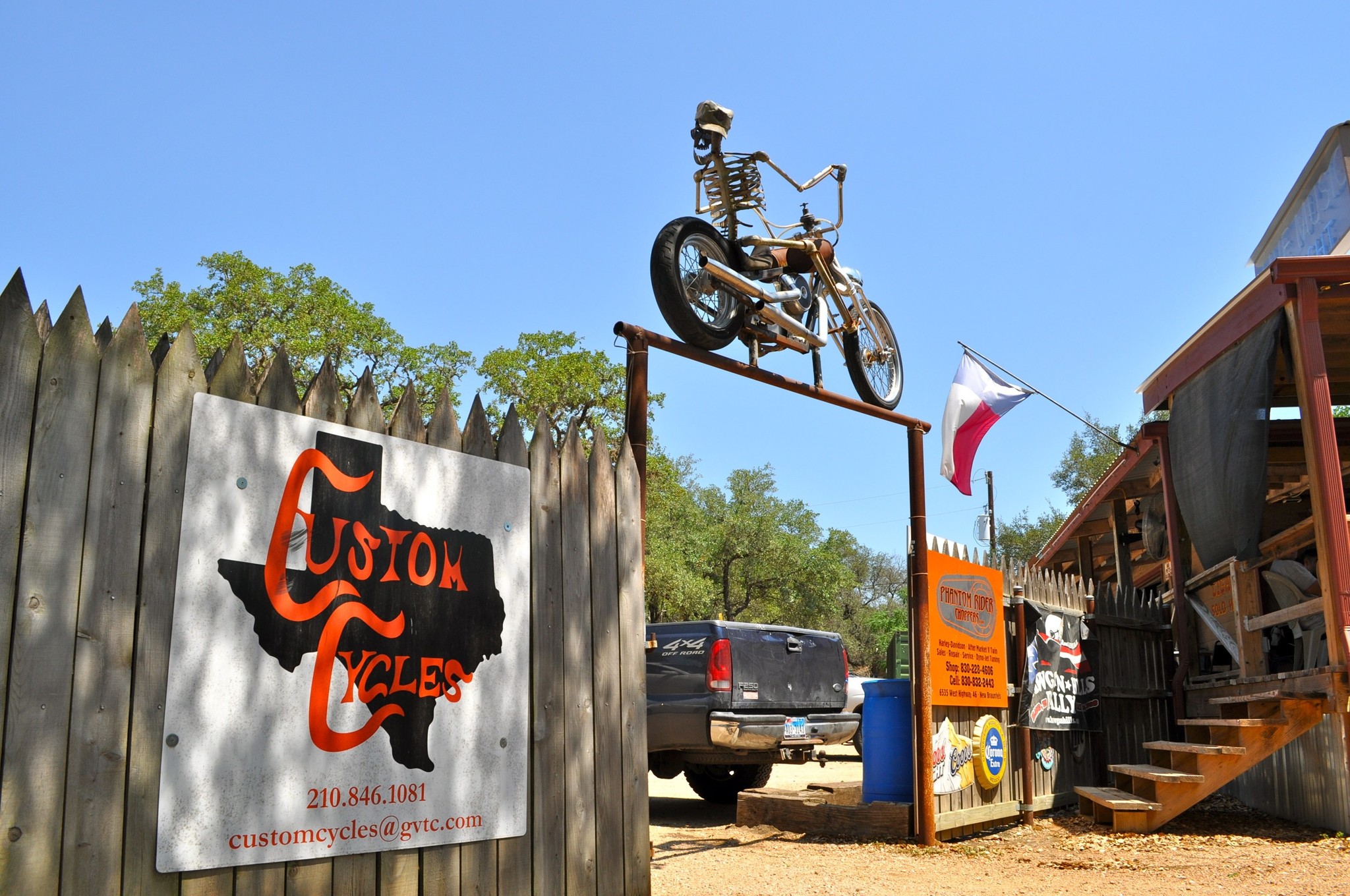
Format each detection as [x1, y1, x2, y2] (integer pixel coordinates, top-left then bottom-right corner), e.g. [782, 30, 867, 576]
[1283, 495, 1302, 504]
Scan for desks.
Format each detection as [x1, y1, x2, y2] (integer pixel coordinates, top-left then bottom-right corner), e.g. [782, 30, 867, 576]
[1196, 650, 1213, 676]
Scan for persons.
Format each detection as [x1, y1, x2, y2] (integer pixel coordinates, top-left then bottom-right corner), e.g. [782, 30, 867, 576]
[1270, 529, 1327, 641]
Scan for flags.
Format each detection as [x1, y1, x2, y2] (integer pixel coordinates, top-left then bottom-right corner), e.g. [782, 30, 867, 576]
[939, 350, 1034, 496]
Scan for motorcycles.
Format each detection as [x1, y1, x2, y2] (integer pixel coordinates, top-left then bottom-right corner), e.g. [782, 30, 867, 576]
[649, 156, 907, 414]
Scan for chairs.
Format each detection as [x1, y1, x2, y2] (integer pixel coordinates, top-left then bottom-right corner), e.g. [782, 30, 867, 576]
[1261, 569, 1329, 672]
[1210, 640, 1232, 674]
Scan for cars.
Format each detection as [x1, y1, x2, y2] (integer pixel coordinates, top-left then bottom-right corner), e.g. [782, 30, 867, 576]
[841, 672, 889, 757]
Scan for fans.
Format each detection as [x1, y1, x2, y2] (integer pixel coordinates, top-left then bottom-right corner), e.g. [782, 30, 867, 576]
[1135, 491, 1167, 562]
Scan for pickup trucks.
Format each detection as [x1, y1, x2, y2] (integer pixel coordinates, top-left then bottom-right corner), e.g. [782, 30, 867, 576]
[643, 620, 860, 804]
